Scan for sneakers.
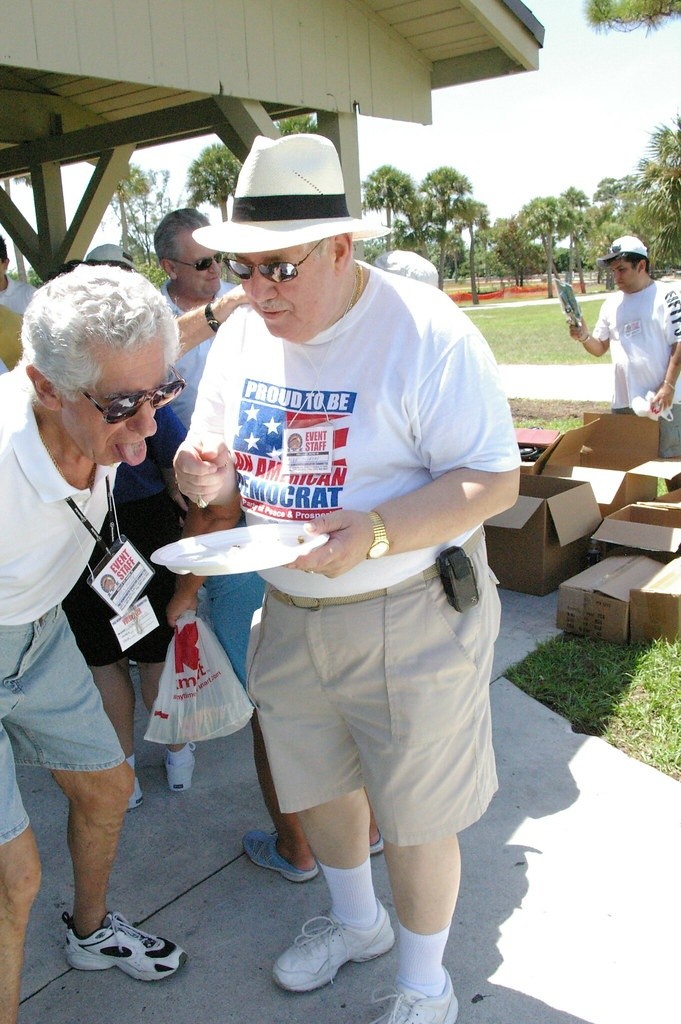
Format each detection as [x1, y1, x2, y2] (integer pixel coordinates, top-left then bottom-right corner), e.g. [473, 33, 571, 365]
[271, 896, 394, 992]
[128, 775, 144, 808]
[62, 910, 188, 981]
[163, 748, 196, 791]
[368, 964, 458, 1024]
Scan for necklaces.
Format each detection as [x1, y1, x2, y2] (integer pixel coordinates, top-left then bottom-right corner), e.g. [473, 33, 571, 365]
[350, 262, 363, 310]
[37, 423, 96, 489]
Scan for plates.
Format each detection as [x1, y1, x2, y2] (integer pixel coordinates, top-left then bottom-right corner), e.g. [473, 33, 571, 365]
[150, 522, 332, 577]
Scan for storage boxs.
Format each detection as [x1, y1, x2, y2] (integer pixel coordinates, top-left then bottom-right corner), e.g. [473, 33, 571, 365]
[483, 413, 681, 646]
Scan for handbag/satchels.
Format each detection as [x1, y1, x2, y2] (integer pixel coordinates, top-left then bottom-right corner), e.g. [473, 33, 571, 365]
[142, 610, 254, 746]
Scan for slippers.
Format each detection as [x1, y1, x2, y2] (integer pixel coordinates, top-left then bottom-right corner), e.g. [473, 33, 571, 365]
[242, 829, 319, 882]
[367, 827, 384, 854]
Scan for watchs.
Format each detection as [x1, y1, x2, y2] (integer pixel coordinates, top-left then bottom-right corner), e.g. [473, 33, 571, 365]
[366, 509, 389, 560]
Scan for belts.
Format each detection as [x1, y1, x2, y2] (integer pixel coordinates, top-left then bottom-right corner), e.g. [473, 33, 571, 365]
[266, 524, 484, 608]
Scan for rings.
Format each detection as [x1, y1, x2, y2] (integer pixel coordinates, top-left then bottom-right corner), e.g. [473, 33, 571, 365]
[304, 569, 314, 573]
[196, 495, 208, 508]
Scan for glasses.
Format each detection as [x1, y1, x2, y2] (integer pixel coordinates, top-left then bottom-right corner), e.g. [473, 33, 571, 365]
[164, 254, 224, 271]
[81, 365, 187, 424]
[223, 238, 322, 282]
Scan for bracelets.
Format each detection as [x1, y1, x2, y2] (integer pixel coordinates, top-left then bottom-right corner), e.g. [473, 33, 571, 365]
[664, 380, 675, 391]
[204, 302, 221, 332]
[579, 334, 591, 343]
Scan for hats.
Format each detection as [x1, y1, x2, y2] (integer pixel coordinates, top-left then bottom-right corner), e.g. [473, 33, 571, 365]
[597, 235, 648, 270]
[373, 249, 440, 290]
[191, 134, 389, 254]
[86, 244, 135, 273]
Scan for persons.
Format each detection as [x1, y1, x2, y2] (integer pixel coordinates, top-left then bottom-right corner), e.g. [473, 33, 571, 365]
[371, 250, 438, 290]
[0, 263, 187, 1024]
[33, 244, 195, 812]
[168, 493, 384, 884]
[569, 235, 681, 494]
[0, 234, 38, 316]
[174, 135, 519, 1024]
[155, 208, 268, 687]
[0, 304, 24, 371]
